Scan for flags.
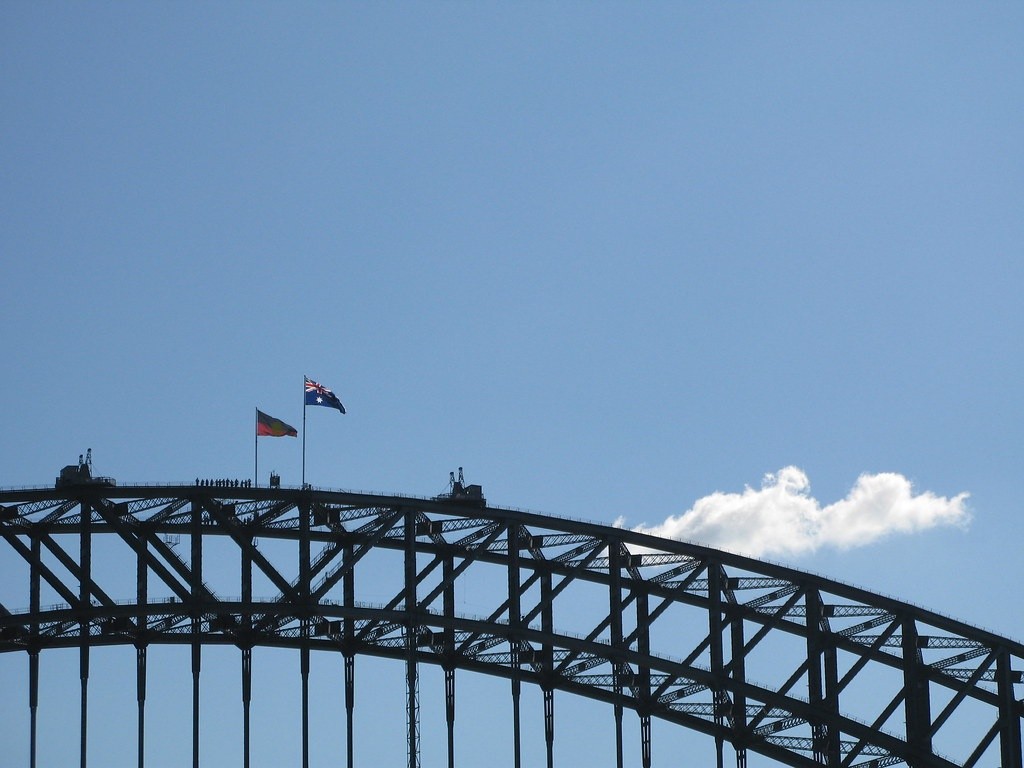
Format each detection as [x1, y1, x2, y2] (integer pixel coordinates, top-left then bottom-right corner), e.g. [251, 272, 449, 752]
[306, 378, 346, 414]
[257, 410, 297, 438]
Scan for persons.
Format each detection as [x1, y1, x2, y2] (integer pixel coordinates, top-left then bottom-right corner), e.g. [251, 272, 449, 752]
[196, 477, 247, 487]
